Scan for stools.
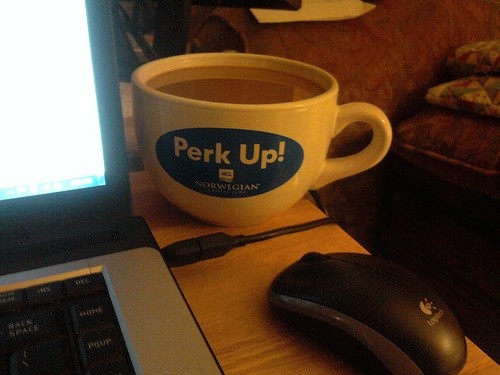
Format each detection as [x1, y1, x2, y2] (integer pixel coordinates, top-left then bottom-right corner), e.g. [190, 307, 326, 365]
[372, 108, 500, 256]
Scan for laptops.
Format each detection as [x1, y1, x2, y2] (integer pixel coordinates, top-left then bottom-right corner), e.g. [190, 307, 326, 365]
[0, 0, 225, 375]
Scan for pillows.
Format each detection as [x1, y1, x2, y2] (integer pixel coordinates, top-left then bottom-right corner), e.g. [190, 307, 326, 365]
[434, 39, 500, 75]
[414, 76, 500, 121]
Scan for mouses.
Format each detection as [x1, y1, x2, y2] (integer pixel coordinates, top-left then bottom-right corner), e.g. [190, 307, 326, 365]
[268, 251, 468, 375]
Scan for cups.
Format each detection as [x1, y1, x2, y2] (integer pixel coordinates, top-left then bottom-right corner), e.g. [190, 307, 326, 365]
[131, 53, 392, 227]
